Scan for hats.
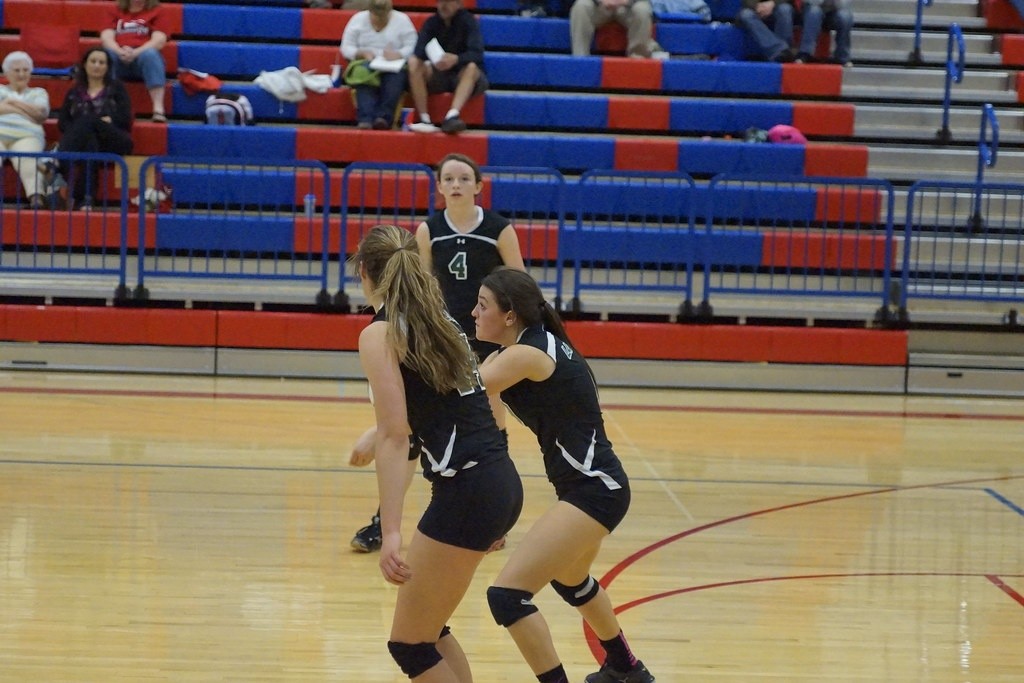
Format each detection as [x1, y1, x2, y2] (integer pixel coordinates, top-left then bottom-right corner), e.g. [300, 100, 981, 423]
[770, 124, 807, 144]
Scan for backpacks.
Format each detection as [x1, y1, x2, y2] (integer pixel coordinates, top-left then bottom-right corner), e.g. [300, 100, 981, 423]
[205, 93, 254, 126]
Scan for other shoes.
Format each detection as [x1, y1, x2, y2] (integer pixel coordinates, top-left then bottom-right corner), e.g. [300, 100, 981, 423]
[31, 195, 44, 208]
[844, 61, 853, 69]
[358, 123, 371, 129]
[80, 202, 93, 210]
[442, 116, 465, 133]
[373, 118, 388, 129]
[773, 48, 796, 63]
[796, 53, 808, 63]
[153, 111, 166, 121]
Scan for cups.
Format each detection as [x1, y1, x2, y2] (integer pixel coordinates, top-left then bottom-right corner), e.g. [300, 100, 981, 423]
[401, 108, 415, 131]
[330, 64, 342, 87]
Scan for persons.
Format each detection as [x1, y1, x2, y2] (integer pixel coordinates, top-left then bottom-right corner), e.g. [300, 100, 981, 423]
[0, 51, 50, 208]
[350, 268, 656, 683]
[705, 0, 853, 68]
[571, 0, 653, 58]
[101, 0, 172, 123]
[57, 48, 132, 211]
[350, 155, 523, 551]
[408, 0, 489, 131]
[352, 223, 524, 682]
[341, 0, 419, 128]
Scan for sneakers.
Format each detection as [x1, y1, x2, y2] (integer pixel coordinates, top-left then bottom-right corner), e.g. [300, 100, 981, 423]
[352, 516, 382, 554]
[584, 660, 654, 683]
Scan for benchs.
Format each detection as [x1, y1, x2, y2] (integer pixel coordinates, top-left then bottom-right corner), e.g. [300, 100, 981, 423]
[1, 0, 1023, 279]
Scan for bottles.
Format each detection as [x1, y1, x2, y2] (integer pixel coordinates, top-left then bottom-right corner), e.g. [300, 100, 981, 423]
[303, 194, 317, 217]
